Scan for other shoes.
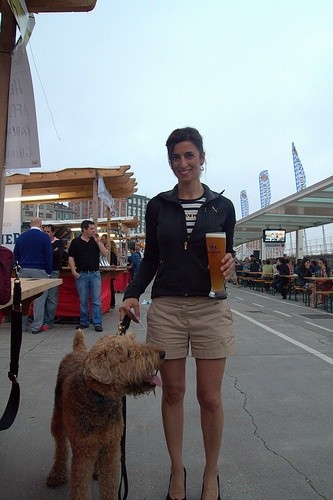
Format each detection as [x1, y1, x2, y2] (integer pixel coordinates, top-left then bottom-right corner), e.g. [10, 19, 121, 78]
[41, 325, 47, 331]
[312, 304, 316, 308]
[318, 303, 323, 306]
[96, 326, 103, 332]
[76, 325, 88, 329]
[32, 330, 39, 334]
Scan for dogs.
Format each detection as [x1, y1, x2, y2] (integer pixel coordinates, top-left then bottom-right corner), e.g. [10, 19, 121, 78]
[46, 328, 167, 500]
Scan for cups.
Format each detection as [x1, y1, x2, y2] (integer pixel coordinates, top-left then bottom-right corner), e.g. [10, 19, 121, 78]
[206, 232, 229, 298]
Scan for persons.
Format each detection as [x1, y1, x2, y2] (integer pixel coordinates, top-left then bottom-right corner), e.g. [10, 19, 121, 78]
[13, 216, 142, 332]
[229, 251, 331, 310]
[119, 127, 237, 500]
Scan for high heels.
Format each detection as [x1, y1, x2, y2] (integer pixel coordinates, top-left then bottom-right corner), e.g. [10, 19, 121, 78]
[201, 475, 220, 500]
[166, 466, 187, 500]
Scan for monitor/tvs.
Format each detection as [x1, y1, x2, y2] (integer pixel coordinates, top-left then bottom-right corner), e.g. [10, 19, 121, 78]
[263, 229, 286, 243]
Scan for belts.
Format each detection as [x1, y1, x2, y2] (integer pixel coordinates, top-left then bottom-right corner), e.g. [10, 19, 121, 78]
[77, 270, 98, 273]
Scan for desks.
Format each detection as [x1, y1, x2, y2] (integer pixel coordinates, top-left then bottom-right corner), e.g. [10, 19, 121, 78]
[303, 276, 333, 313]
[0, 278, 63, 310]
[237, 270, 297, 300]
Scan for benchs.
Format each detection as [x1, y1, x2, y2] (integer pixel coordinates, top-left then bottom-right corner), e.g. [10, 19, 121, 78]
[237, 276, 333, 308]
